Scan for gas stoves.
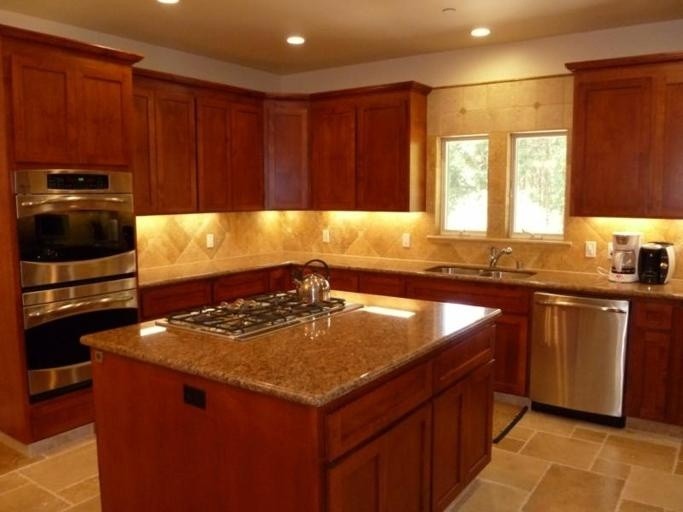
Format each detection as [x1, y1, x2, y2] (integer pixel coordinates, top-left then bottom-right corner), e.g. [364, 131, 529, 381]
[154, 288, 366, 344]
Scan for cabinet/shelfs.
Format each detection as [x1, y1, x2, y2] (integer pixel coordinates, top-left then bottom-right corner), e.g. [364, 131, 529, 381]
[1, 23, 144, 169]
[90, 325, 495, 511]
[495, 283, 528, 395]
[311, 79, 432, 212]
[263, 92, 308, 211]
[628, 297, 682, 426]
[133, 67, 196, 216]
[196, 78, 264, 214]
[570, 67, 682, 219]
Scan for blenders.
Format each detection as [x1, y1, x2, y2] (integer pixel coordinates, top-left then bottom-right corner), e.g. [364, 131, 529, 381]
[607, 232, 640, 285]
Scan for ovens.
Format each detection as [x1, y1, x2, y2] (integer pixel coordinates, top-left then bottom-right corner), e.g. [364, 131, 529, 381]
[12, 168, 142, 406]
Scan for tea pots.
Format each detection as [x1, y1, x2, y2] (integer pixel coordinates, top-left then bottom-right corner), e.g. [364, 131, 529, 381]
[290, 258, 331, 306]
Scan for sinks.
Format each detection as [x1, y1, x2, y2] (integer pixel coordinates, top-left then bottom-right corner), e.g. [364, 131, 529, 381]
[423, 264, 488, 277]
[478, 270, 537, 281]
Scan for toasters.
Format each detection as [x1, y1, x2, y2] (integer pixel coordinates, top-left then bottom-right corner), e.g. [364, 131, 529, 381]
[636, 241, 676, 284]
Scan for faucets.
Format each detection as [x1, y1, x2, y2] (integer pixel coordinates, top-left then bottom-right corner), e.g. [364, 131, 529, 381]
[489, 247, 513, 268]
